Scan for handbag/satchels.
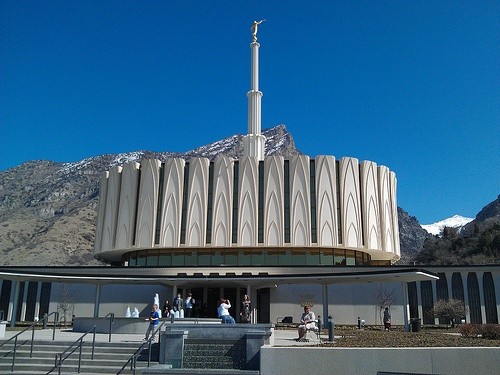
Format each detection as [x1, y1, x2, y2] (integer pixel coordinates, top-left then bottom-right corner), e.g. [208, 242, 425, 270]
[190, 298, 196, 304]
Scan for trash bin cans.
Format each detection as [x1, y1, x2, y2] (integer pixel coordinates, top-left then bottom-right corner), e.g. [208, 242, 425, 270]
[411, 318, 422, 333]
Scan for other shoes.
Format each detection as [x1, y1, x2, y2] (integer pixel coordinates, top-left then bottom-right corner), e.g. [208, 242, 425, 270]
[142, 337, 156, 341]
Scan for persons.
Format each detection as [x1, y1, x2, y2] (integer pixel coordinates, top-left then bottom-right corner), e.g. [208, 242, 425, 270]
[173, 294, 184, 318]
[240, 294, 252, 323]
[298, 305, 315, 339]
[163, 301, 171, 318]
[383, 308, 391, 333]
[218, 298, 236, 324]
[142, 304, 159, 342]
[250, 20, 263, 36]
[184, 293, 193, 318]
